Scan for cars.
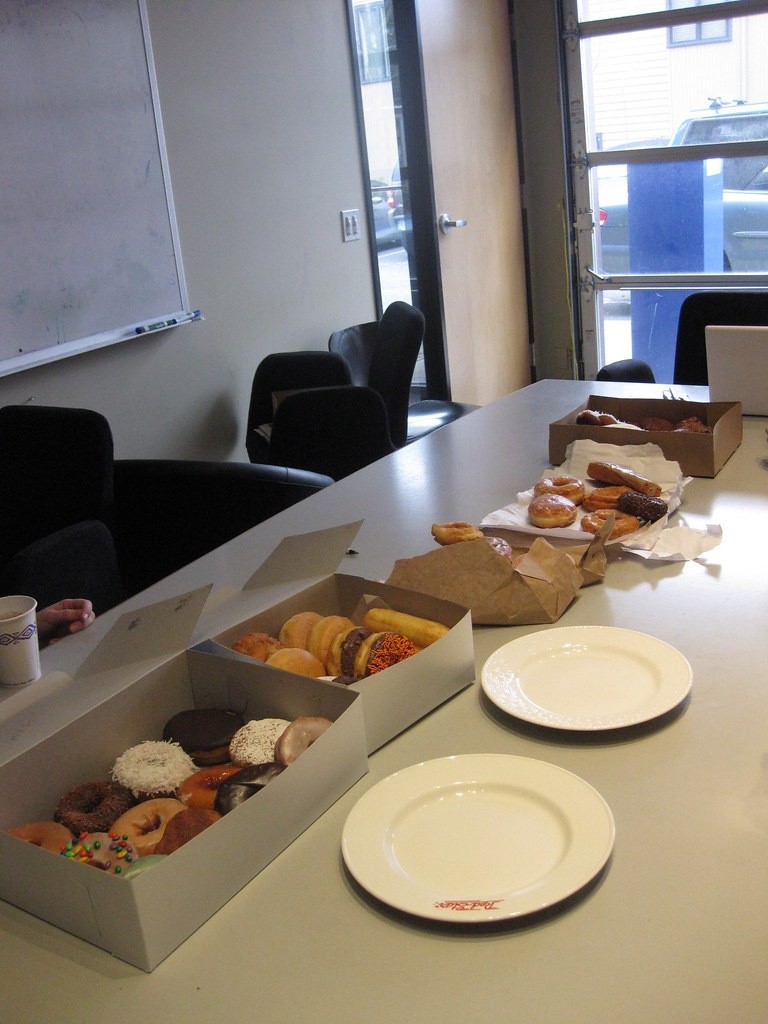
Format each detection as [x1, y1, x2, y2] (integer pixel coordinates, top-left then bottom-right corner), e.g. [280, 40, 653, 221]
[370, 180, 402, 247]
[589, 138, 669, 318]
[668, 101, 768, 270]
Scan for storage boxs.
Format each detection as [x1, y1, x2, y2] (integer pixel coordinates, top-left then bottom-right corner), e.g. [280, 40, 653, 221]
[549, 394, 743, 478]
[0, 572, 478, 975]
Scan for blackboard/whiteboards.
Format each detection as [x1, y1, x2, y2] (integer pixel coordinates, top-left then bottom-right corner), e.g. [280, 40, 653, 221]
[0, 1, 206, 378]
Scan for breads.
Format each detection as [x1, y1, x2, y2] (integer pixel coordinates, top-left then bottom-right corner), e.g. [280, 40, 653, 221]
[230, 608, 355, 679]
[229, 718, 291, 765]
[575, 409, 714, 434]
[528, 461, 662, 539]
[364, 608, 450, 651]
[165, 708, 244, 766]
[431, 523, 528, 571]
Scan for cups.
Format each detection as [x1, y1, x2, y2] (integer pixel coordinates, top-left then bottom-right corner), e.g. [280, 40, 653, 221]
[0, 595, 41, 689]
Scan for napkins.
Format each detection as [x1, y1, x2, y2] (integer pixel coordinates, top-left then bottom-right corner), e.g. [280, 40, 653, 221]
[483, 439, 724, 566]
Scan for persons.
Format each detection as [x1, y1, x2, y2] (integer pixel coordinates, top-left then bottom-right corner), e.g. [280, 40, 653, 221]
[36, 598, 95, 649]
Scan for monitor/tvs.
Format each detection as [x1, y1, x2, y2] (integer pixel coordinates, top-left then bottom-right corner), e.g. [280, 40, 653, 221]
[706, 325, 768, 416]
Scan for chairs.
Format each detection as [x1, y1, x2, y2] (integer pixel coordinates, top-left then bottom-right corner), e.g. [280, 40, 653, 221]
[671, 291, 768, 386]
[0, 300, 482, 651]
[593, 358, 656, 383]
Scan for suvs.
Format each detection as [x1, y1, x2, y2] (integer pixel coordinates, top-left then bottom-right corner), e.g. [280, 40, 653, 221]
[388, 163, 405, 250]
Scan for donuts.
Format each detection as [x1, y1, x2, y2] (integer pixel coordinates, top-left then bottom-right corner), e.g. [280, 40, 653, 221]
[315, 625, 419, 685]
[0, 707, 334, 880]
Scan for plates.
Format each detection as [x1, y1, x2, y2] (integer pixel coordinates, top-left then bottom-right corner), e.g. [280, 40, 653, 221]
[481, 626, 694, 730]
[341, 753, 615, 923]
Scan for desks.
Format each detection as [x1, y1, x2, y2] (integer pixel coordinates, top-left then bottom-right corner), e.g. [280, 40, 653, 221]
[0, 379, 768, 1024]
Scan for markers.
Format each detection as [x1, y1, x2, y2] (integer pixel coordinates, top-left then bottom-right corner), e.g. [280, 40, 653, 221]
[172, 310, 201, 323]
[134, 320, 173, 334]
